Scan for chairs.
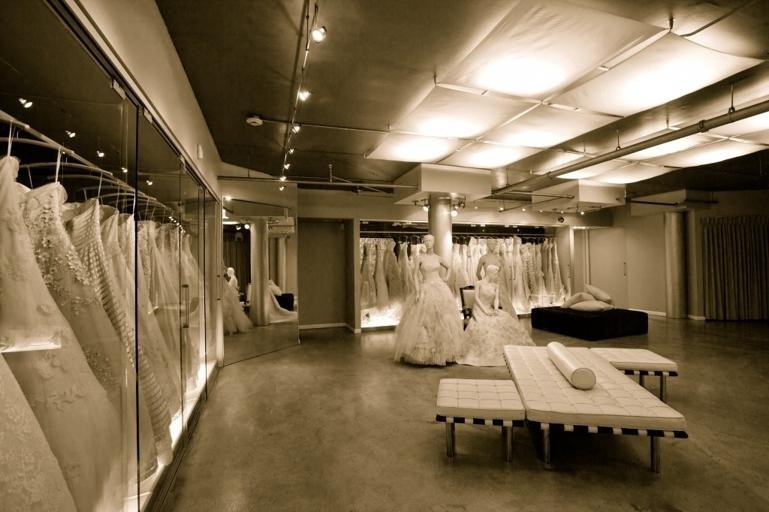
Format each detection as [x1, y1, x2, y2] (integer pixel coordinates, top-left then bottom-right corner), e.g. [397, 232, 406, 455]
[459, 286, 481, 330]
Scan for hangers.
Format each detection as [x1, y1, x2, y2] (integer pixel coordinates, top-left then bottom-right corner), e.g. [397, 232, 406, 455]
[0, 111, 174, 222]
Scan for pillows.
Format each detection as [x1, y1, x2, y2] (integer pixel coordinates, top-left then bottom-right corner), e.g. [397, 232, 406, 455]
[561, 281, 614, 312]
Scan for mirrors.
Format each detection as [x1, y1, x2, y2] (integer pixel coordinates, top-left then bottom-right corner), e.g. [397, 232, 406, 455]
[219, 195, 301, 368]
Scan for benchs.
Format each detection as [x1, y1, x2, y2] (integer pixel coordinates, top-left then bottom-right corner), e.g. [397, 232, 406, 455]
[503, 344, 689, 473]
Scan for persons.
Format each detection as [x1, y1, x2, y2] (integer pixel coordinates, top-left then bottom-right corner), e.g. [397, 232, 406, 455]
[476, 240, 518, 321]
[227, 267, 238, 289]
[456, 265, 536, 368]
[393, 236, 464, 367]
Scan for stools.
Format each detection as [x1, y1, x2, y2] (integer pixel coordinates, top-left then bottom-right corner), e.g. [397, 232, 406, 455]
[437, 379, 526, 476]
[589, 346, 678, 405]
